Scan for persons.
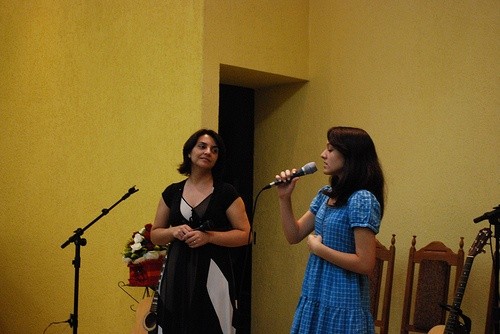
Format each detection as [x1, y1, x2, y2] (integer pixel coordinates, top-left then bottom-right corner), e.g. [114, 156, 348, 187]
[274, 125, 385, 334]
[151, 129, 252, 334]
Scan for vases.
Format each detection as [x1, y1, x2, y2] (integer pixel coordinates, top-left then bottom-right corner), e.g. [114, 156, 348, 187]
[127, 260, 163, 285]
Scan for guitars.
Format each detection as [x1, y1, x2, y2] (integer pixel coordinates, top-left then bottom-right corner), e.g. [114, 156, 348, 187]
[130, 240, 170, 334]
[428, 227, 493, 334]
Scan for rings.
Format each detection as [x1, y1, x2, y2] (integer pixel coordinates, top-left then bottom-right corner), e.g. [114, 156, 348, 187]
[194, 240, 197, 244]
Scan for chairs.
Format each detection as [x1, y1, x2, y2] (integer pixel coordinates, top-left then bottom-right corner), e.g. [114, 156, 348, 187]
[400, 236, 465, 334]
[367, 234, 396, 334]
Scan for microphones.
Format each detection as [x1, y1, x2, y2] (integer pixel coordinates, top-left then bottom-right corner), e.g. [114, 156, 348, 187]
[262, 162, 318, 190]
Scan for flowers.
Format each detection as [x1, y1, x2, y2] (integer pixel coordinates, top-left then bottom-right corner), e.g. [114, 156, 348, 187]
[124, 224, 167, 264]
